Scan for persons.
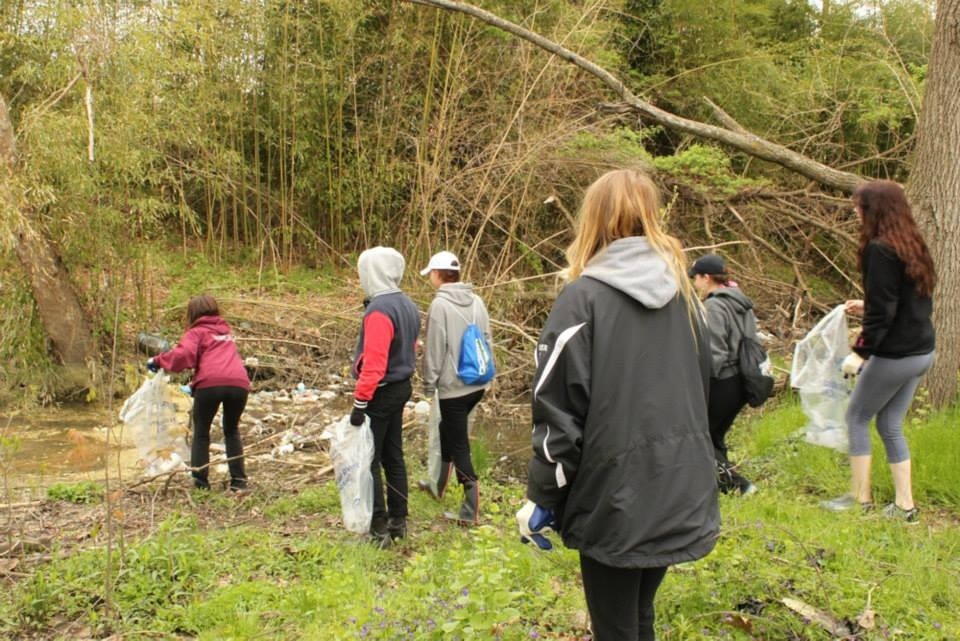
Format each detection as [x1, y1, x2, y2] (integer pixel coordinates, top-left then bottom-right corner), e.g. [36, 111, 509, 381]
[819, 182, 937, 522]
[350, 247, 422, 547]
[416, 250, 492, 524]
[517, 170, 722, 641]
[147, 294, 249, 492]
[686, 254, 759, 499]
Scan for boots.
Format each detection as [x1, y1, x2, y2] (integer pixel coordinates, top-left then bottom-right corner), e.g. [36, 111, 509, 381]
[416, 462, 452, 499]
[444, 480, 478, 528]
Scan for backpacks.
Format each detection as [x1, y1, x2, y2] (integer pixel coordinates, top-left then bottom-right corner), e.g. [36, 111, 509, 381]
[437, 297, 494, 387]
[721, 300, 774, 407]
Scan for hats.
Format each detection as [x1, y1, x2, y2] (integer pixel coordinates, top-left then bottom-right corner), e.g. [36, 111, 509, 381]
[420, 251, 461, 275]
[686, 254, 727, 277]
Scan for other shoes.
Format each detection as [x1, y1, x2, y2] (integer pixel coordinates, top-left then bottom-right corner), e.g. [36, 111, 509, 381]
[387, 519, 404, 543]
[221, 477, 249, 492]
[737, 480, 757, 499]
[190, 480, 211, 492]
[359, 529, 391, 550]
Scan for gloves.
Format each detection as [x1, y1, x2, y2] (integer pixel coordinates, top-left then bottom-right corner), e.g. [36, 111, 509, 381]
[147, 358, 159, 372]
[515, 500, 553, 550]
[350, 406, 365, 425]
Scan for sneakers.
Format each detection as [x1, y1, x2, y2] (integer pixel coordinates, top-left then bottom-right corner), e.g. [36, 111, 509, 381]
[820, 494, 873, 512]
[876, 504, 919, 526]
